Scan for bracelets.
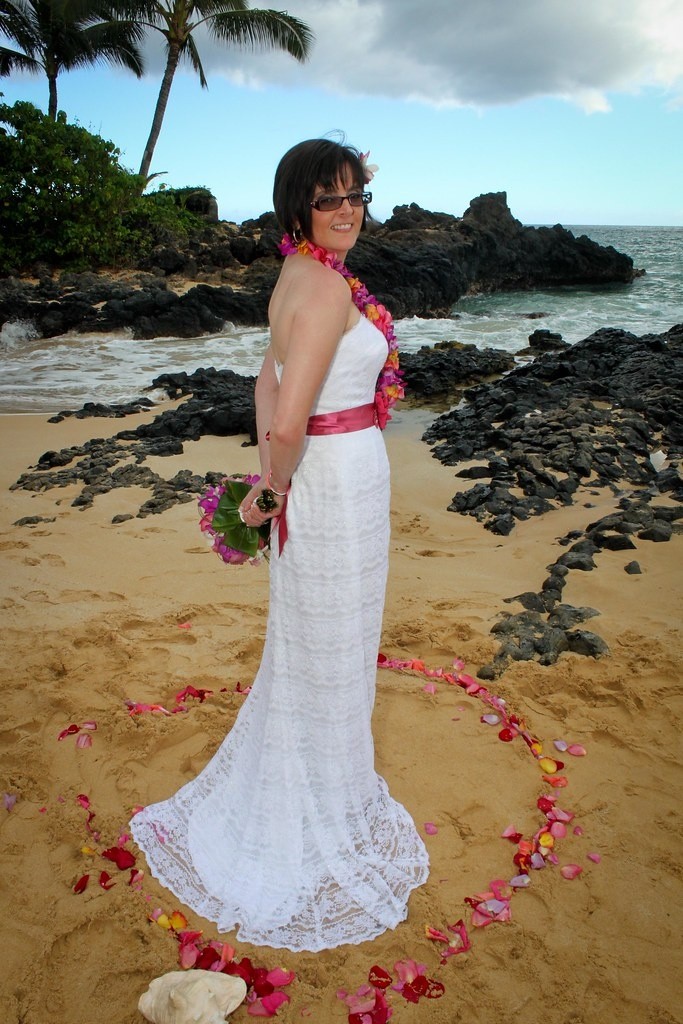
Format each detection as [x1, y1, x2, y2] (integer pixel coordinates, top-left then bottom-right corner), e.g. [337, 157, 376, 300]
[267, 476, 287, 497]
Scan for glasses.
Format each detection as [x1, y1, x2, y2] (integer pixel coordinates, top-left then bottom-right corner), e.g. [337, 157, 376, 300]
[303, 191, 372, 211]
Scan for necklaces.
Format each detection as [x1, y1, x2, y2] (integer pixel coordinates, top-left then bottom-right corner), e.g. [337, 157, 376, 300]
[277, 233, 407, 431]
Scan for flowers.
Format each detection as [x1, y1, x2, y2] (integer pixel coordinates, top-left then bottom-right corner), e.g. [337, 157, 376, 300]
[198, 473, 279, 567]
[358, 150, 379, 184]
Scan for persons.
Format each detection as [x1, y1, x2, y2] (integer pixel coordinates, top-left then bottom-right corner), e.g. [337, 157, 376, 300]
[127, 137, 430, 954]
